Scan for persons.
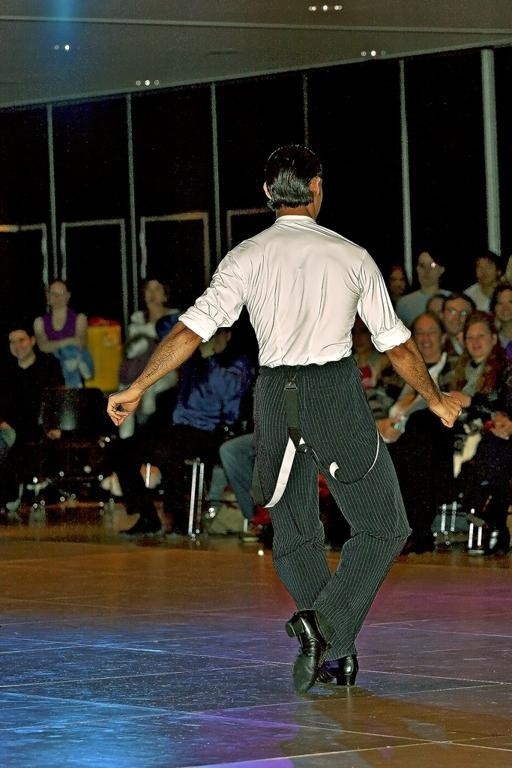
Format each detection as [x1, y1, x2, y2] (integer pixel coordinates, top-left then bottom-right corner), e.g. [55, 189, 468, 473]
[33, 278, 87, 353]
[112, 327, 253, 540]
[104, 145, 463, 695]
[1, 325, 64, 510]
[124, 278, 180, 357]
[352, 250, 511, 553]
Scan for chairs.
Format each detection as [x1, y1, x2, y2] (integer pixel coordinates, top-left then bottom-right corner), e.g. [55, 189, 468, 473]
[0, 387, 511, 555]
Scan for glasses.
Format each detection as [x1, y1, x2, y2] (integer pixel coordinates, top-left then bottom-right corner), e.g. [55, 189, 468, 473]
[446, 306, 469, 317]
[420, 260, 438, 269]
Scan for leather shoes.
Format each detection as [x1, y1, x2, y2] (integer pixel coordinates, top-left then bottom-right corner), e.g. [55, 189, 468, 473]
[400, 534, 438, 556]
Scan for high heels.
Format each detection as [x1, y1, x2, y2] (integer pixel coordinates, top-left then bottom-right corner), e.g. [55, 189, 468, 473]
[468, 528, 510, 558]
[284, 608, 325, 695]
[314, 656, 361, 687]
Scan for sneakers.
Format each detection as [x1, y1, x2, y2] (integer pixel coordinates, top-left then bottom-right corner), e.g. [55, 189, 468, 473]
[117, 511, 162, 539]
[165, 517, 200, 536]
